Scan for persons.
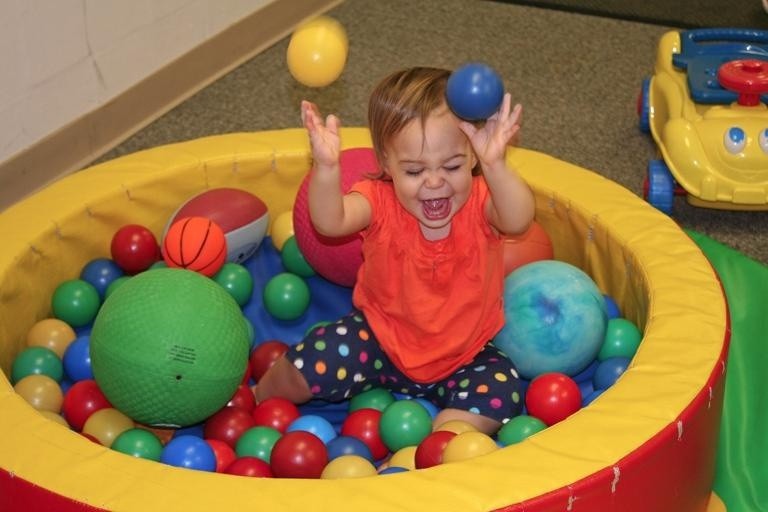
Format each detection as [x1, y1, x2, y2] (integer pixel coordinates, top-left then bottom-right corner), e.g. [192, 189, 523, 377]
[248, 68, 537, 433]
